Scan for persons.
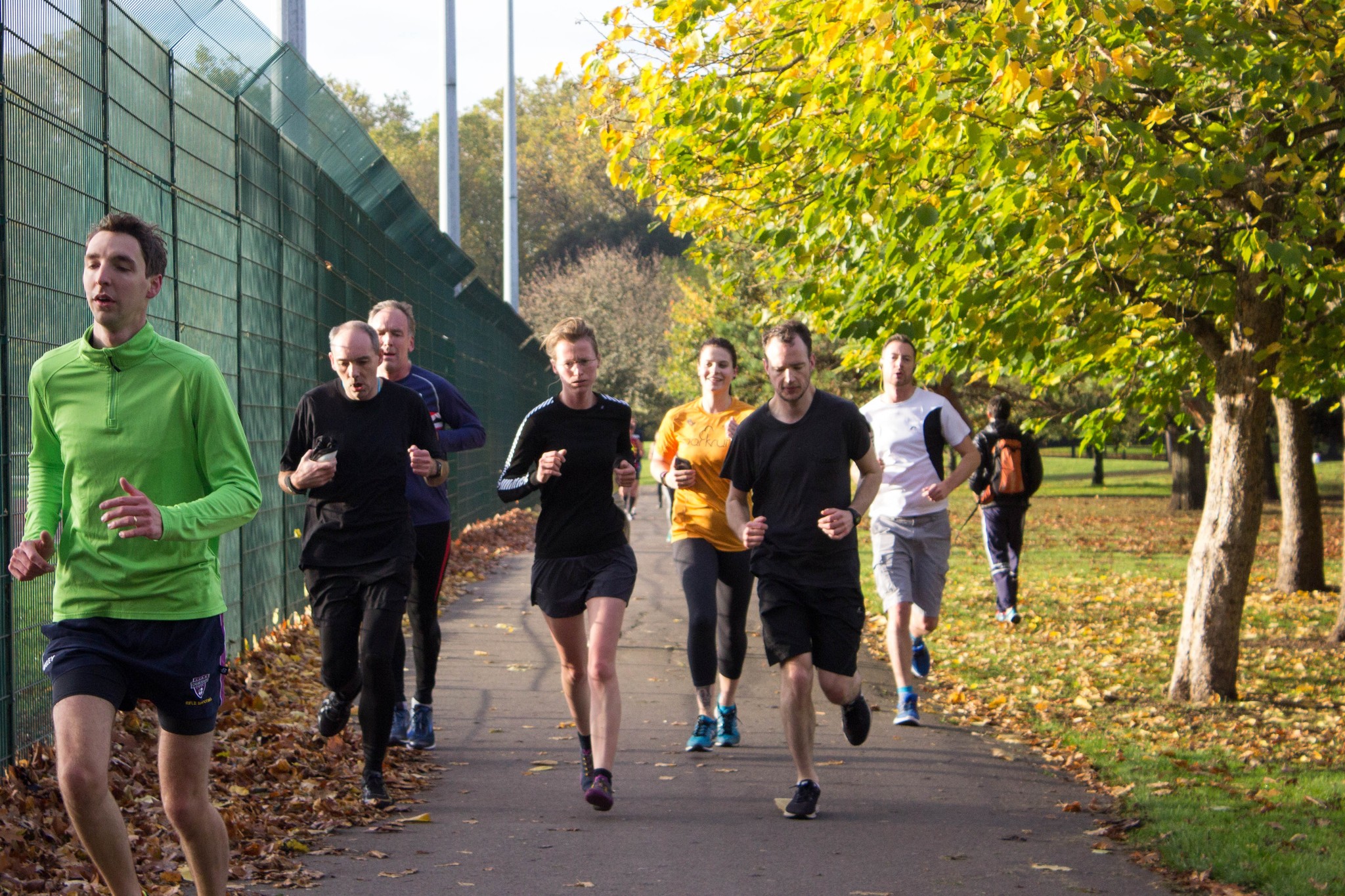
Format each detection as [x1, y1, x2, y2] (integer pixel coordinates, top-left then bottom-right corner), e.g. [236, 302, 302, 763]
[968, 395, 1043, 622]
[8, 213, 262, 896]
[650, 338, 756, 752]
[648, 432, 675, 543]
[368, 301, 485, 748]
[276, 321, 449, 808]
[620, 422, 644, 521]
[719, 321, 882, 819]
[851, 335, 982, 725]
[497, 318, 639, 810]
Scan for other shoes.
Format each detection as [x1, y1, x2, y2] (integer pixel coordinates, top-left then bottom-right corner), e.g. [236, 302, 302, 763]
[996, 608, 1020, 625]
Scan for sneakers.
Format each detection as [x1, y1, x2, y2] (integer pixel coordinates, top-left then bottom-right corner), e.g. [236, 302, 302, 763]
[686, 716, 717, 752]
[360, 774, 394, 808]
[892, 688, 920, 725]
[716, 692, 744, 748]
[840, 694, 871, 747]
[317, 671, 363, 737]
[585, 776, 613, 812]
[580, 749, 595, 793]
[409, 706, 436, 749]
[783, 779, 821, 819]
[389, 708, 412, 745]
[909, 637, 929, 679]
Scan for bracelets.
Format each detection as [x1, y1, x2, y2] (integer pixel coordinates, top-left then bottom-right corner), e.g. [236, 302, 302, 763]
[284, 475, 307, 495]
[660, 471, 668, 486]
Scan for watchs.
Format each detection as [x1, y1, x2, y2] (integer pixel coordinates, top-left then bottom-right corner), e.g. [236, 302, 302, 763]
[844, 507, 861, 526]
[427, 460, 443, 478]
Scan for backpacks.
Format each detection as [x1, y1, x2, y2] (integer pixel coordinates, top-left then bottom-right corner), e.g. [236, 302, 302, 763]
[982, 429, 1024, 501]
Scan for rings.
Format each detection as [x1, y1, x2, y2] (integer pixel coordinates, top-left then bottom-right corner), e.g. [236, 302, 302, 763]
[134, 516, 137, 525]
